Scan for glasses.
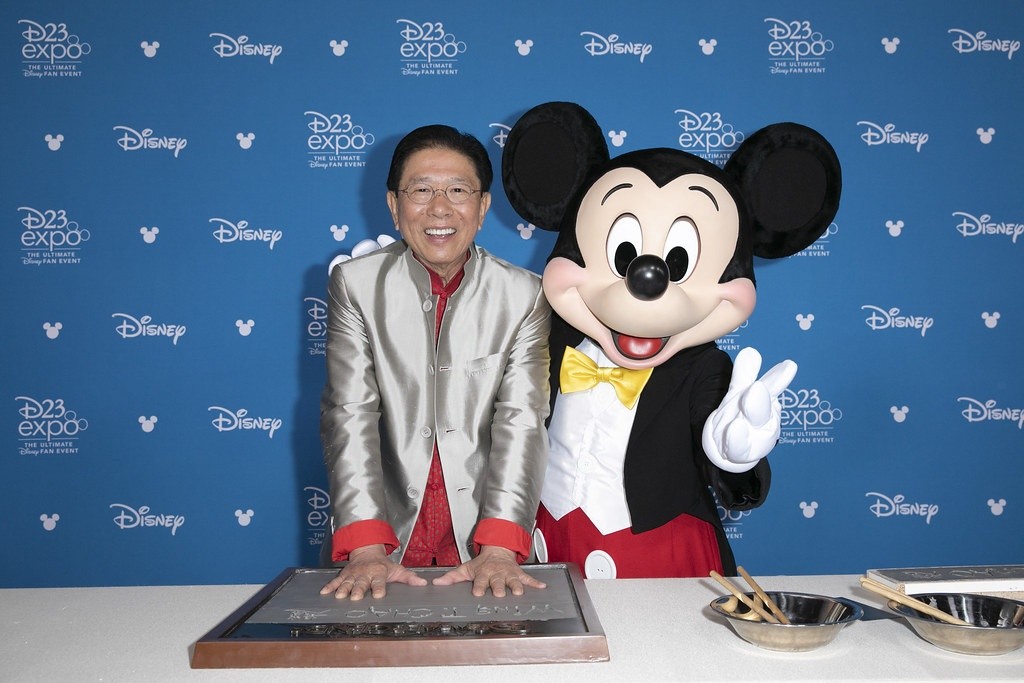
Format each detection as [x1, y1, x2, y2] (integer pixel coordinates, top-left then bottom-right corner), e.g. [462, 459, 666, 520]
[396, 183, 481, 204]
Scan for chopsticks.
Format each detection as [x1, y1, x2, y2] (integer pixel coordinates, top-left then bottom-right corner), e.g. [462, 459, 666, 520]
[710, 565, 791, 625]
[860, 577, 970, 626]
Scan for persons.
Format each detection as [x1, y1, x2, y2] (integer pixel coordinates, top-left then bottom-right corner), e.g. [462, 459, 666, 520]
[318, 126, 552, 602]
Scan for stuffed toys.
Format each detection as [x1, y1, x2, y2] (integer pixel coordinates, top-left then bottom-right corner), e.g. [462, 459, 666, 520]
[326, 102, 842, 578]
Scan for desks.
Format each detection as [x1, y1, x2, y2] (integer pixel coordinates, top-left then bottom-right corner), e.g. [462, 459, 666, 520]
[1, 571, 1022, 681]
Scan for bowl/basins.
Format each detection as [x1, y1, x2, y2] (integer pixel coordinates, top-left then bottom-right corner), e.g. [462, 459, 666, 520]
[887, 593, 1024, 656]
[710, 590, 864, 652]
